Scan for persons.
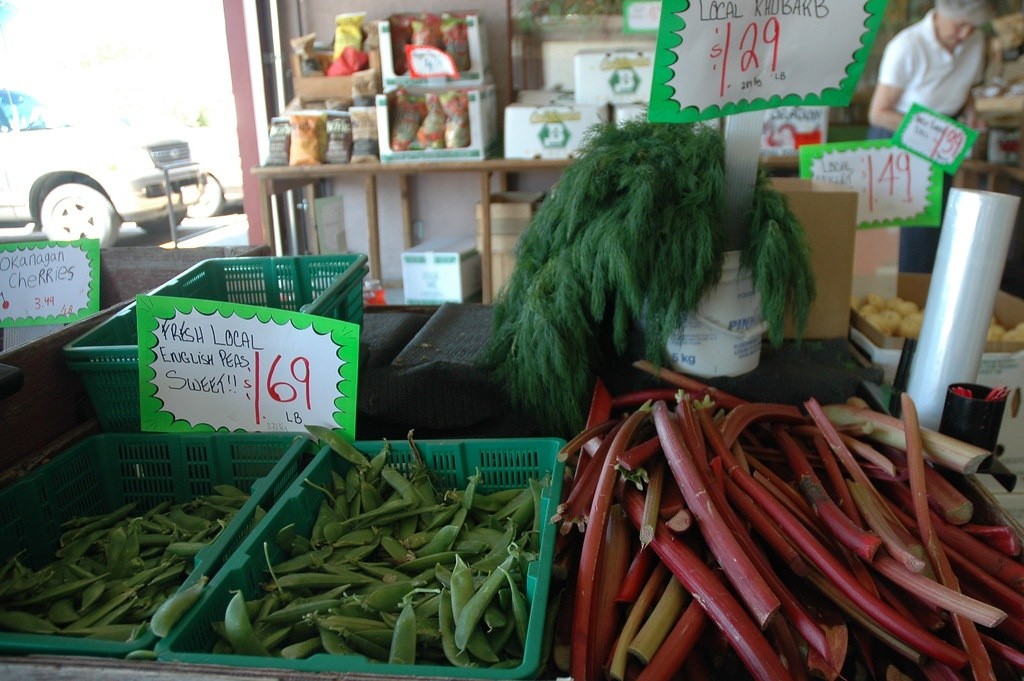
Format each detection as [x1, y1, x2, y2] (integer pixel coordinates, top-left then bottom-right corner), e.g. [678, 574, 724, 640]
[868, 0, 997, 275]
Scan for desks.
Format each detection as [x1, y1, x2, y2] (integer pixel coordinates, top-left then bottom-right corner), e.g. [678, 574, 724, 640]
[247, 157, 577, 303]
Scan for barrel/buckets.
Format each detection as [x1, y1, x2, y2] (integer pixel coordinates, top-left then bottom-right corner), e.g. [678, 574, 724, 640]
[665, 249, 770, 378]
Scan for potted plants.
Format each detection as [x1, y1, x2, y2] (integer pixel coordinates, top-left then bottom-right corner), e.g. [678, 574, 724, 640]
[476, 105, 816, 440]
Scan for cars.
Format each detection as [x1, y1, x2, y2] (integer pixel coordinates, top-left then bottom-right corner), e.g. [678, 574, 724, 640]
[119, 102, 270, 220]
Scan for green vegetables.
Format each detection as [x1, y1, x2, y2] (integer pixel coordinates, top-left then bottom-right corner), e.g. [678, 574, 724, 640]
[474, 106, 816, 442]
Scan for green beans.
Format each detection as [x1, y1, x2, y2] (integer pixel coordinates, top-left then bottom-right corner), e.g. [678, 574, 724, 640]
[211, 424, 551, 673]
[0, 483, 267, 660]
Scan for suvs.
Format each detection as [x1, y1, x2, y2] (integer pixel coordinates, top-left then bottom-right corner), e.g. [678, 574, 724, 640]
[0, 89, 210, 250]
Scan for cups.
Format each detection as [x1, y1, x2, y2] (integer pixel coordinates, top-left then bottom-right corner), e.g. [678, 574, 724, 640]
[937, 382, 1005, 472]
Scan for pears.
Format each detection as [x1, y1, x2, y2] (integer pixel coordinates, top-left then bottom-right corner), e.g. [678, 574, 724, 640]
[850, 291, 1024, 341]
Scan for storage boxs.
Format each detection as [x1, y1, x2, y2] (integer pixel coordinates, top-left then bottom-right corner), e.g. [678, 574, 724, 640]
[377, 9, 490, 85]
[482, 253, 518, 306]
[766, 176, 858, 341]
[475, 190, 545, 253]
[504, 104, 608, 160]
[574, 46, 656, 106]
[290, 46, 382, 101]
[760, 105, 830, 159]
[850, 272, 1024, 494]
[401, 235, 480, 304]
[611, 106, 720, 137]
[0, 437, 569, 681]
[375, 84, 497, 164]
[60, 253, 369, 429]
